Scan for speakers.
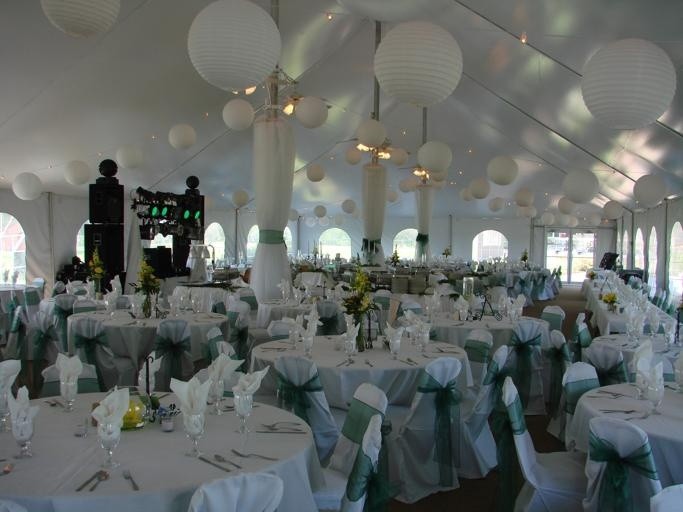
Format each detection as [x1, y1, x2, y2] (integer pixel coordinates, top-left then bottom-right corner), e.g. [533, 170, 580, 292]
[84, 224, 124, 273]
[176, 194, 204, 228]
[172, 227, 204, 268]
[88, 184, 124, 224]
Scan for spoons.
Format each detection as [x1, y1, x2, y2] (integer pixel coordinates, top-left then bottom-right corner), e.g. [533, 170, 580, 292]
[214, 454, 241, 469]
[89, 472, 109, 491]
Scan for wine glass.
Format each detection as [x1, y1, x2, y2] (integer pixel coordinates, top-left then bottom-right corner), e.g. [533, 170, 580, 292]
[183, 413, 208, 458]
[208, 381, 224, 416]
[649, 385, 664, 416]
[98, 423, 121, 467]
[11, 419, 34, 460]
[233, 394, 254, 432]
[0, 393, 11, 433]
[60, 380, 78, 413]
[674, 369, 683, 394]
[635, 371, 649, 401]
[0, 250, 683, 364]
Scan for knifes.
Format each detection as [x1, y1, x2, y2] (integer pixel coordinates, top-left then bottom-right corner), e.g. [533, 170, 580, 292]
[198, 457, 230, 473]
[76, 470, 102, 492]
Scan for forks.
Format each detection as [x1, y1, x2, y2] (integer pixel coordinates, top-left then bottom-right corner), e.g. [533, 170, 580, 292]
[232, 449, 277, 461]
[122, 469, 140, 491]
